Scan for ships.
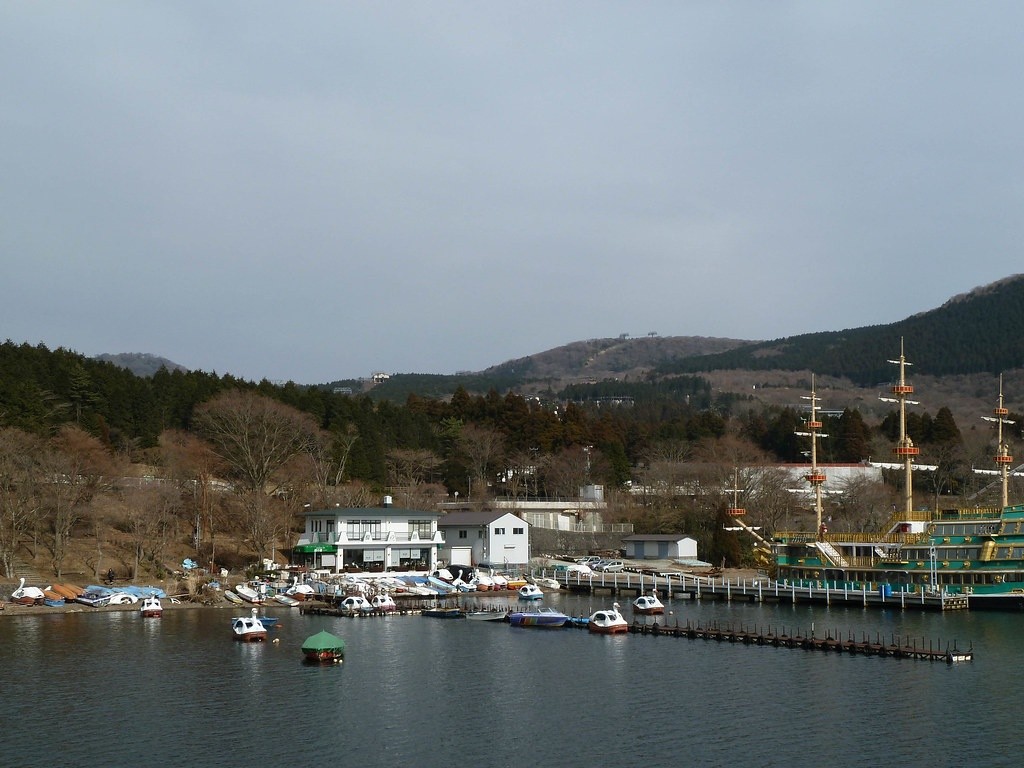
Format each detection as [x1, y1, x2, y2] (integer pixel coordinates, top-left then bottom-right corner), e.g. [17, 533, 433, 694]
[720, 334, 1024, 606]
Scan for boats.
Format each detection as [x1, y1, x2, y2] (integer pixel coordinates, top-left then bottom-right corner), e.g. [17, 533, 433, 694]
[452, 567, 508, 592]
[633, 588, 665, 616]
[230, 606, 280, 643]
[10, 577, 45, 604]
[139, 591, 164, 617]
[427, 575, 456, 592]
[420, 602, 569, 627]
[286, 576, 315, 596]
[274, 593, 300, 607]
[224, 583, 259, 604]
[518, 579, 544, 601]
[340, 587, 397, 614]
[586, 601, 629, 635]
[232, 617, 279, 626]
[299, 627, 347, 664]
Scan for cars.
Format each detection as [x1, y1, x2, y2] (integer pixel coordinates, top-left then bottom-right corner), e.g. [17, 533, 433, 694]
[577, 556, 625, 573]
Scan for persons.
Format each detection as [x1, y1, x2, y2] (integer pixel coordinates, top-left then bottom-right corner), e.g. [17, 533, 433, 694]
[818, 522, 828, 543]
[979, 525, 995, 535]
[108, 568, 114, 585]
